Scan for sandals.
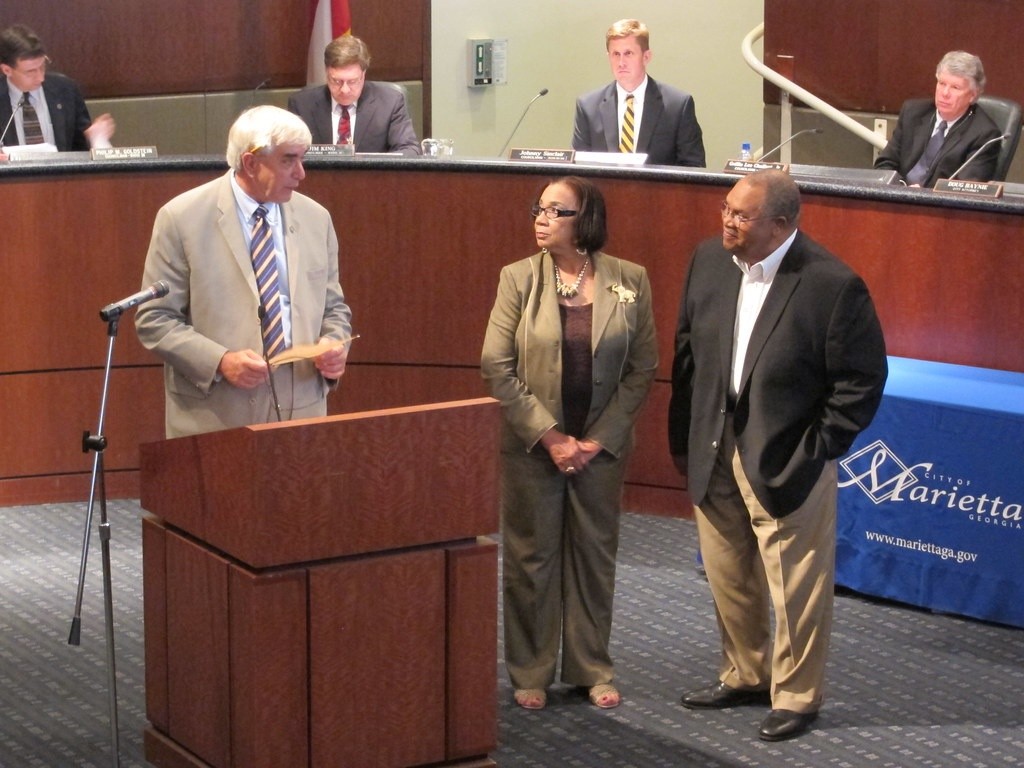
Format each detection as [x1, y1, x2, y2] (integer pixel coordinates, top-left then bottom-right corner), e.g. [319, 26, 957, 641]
[513, 688, 546, 709]
[587, 682, 620, 709]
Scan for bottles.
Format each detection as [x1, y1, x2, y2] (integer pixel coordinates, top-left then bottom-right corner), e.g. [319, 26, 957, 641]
[738, 144, 754, 161]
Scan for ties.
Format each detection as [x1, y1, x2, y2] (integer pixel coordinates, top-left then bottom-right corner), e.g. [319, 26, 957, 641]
[22, 91, 45, 146]
[619, 94, 634, 154]
[905, 120, 947, 186]
[336, 103, 351, 144]
[250, 205, 287, 370]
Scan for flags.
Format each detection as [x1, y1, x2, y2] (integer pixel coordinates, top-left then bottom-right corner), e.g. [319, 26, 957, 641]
[307, 0, 352, 88]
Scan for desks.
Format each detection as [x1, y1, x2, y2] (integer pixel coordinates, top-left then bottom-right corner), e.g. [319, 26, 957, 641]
[698, 356, 1023, 629]
[1, 151, 1023, 517]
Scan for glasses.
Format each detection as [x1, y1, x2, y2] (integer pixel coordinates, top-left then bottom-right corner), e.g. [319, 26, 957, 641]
[15, 53, 52, 78]
[328, 69, 364, 86]
[720, 200, 779, 227]
[529, 204, 580, 220]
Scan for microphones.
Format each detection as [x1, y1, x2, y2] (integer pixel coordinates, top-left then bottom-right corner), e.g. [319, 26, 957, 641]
[252, 79, 272, 106]
[497, 88, 548, 157]
[258, 305, 281, 422]
[757, 128, 824, 162]
[99, 279, 170, 322]
[948, 133, 1011, 180]
[0, 97, 26, 142]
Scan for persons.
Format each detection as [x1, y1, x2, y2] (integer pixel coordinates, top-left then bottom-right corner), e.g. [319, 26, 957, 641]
[571, 19, 707, 167]
[288, 34, 421, 156]
[668, 169, 889, 742]
[873, 50, 1001, 187]
[479, 175, 659, 709]
[135, 104, 352, 439]
[0, 24, 115, 151]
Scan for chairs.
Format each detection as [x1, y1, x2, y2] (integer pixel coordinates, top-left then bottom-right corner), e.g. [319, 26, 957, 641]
[977, 95, 1024, 182]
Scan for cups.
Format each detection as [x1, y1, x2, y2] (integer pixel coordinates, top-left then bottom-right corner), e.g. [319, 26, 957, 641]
[421, 138, 454, 156]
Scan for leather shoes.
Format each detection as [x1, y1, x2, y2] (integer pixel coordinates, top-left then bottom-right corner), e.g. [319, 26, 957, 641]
[681, 680, 772, 710]
[757, 706, 819, 741]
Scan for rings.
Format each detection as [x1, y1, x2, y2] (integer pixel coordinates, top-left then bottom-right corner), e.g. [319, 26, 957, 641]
[565, 466, 575, 474]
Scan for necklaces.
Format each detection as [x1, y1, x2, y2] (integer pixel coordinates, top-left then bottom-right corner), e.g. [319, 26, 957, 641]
[554, 258, 589, 299]
